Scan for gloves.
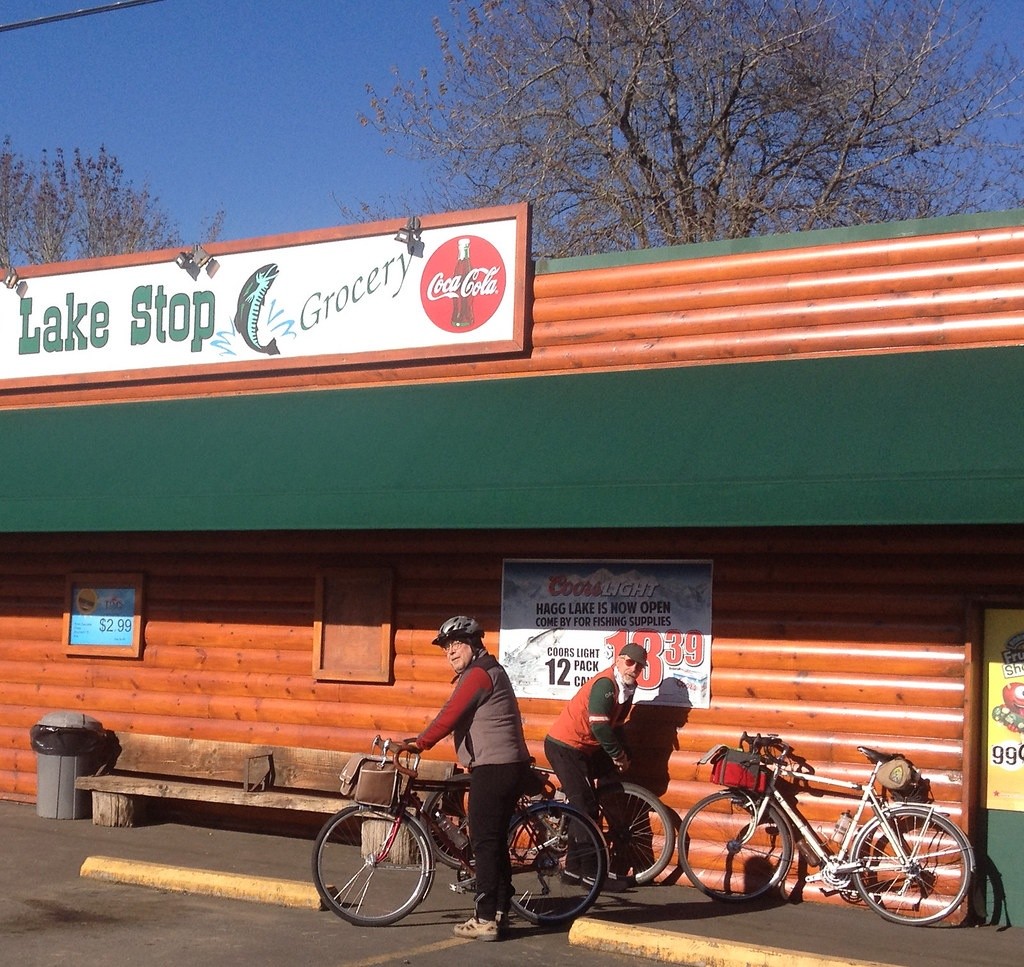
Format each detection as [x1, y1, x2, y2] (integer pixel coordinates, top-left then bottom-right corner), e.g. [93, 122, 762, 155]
[613, 752, 630, 773]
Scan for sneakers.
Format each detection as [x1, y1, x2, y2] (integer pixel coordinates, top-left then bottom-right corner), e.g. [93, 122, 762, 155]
[583, 871, 629, 892]
[495, 911, 509, 930]
[563, 869, 597, 885]
[454, 917, 500, 942]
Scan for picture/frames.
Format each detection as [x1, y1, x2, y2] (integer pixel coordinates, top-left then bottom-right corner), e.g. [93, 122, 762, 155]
[62, 573, 144, 658]
[312, 568, 394, 683]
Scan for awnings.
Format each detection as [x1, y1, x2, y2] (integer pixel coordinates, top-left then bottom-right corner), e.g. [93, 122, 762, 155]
[0, 345, 1024, 532]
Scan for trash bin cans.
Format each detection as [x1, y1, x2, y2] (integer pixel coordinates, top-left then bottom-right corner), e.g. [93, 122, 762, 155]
[31, 710, 104, 820]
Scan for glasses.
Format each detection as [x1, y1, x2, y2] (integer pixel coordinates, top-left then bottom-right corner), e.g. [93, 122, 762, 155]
[443, 640, 467, 653]
[620, 656, 643, 671]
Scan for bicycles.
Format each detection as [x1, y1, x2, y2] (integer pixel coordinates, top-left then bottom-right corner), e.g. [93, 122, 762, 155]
[312, 734, 610, 926]
[420, 756, 675, 889]
[678, 731, 976, 926]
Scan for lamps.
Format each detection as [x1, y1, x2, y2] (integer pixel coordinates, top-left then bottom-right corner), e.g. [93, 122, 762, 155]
[3, 266, 21, 288]
[174, 241, 212, 270]
[395, 217, 422, 244]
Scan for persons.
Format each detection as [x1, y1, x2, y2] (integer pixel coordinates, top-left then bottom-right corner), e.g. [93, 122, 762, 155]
[401, 616, 531, 941]
[546, 644, 649, 893]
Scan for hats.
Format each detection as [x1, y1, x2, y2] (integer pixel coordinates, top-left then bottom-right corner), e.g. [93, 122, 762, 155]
[619, 643, 648, 667]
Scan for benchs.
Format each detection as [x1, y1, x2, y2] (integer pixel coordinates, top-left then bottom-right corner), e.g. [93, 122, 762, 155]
[74, 730, 457, 870]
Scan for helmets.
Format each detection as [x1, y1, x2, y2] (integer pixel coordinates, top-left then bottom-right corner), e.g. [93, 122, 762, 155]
[432, 615, 484, 645]
